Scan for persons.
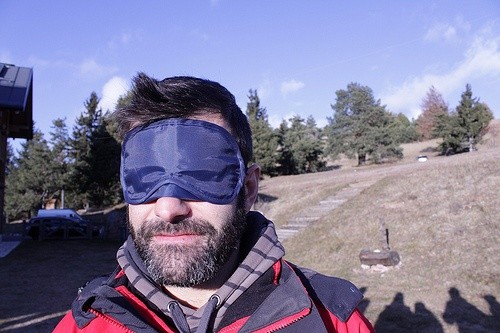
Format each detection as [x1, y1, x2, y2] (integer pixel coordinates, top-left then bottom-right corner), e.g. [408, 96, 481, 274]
[44, 76, 375, 332]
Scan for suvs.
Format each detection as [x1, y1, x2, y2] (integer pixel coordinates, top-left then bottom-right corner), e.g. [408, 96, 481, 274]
[26, 216, 104, 237]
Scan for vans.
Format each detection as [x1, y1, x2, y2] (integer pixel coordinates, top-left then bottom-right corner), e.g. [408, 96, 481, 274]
[37, 208, 84, 222]
[417, 154, 428, 162]
